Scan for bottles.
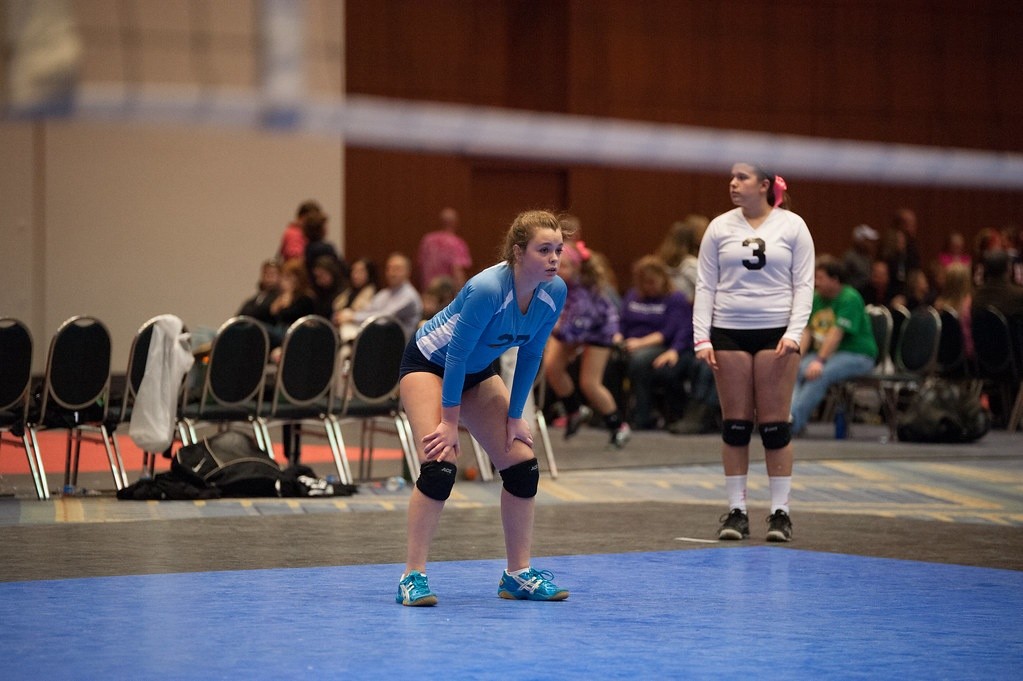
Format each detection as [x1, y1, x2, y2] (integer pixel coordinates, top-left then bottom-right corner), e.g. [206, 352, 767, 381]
[835, 413, 847, 437]
[386, 476, 405, 491]
[57, 485, 87, 497]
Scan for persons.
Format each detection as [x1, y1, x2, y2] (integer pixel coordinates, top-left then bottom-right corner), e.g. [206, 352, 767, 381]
[395, 202, 567, 603]
[789, 205, 1023, 438]
[694, 161, 815, 542]
[415, 204, 471, 291]
[544, 217, 632, 447]
[423, 275, 457, 315]
[236, 197, 423, 402]
[605, 214, 712, 432]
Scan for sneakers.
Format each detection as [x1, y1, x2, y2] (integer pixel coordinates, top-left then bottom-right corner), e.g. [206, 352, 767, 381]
[764, 509, 794, 542]
[497, 568, 570, 600]
[395, 572, 439, 606]
[714, 507, 751, 539]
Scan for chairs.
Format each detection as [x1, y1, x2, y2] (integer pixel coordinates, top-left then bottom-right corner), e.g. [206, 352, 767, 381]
[0, 314, 556, 499]
[813, 299, 1023, 445]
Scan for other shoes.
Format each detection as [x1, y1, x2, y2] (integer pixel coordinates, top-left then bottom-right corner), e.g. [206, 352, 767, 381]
[561, 406, 591, 442]
[603, 422, 632, 452]
